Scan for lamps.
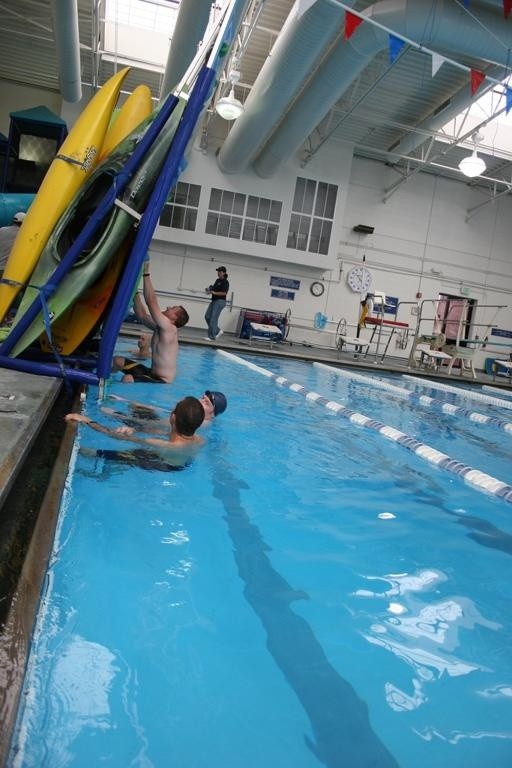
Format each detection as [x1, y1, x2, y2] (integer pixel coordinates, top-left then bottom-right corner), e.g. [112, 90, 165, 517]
[214, 58, 246, 120]
[458, 133, 486, 178]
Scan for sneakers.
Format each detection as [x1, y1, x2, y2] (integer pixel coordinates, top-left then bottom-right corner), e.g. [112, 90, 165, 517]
[203, 330, 224, 342]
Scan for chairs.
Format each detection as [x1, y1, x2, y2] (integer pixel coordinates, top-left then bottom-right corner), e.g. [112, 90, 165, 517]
[375, 291, 396, 321]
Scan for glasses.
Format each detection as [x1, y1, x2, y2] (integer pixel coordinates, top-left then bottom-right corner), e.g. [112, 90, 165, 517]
[205, 390, 213, 406]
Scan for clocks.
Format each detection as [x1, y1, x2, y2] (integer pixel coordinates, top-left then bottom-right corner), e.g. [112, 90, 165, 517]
[311, 282, 324, 296]
[348, 267, 372, 293]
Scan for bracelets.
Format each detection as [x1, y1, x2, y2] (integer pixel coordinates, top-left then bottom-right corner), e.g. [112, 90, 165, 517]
[144, 272, 150, 277]
[85, 420, 98, 425]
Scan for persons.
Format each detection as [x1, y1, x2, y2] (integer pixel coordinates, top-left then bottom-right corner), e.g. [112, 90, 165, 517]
[111, 261, 190, 383]
[112, 332, 153, 358]
[62, 395, 207, 484]
[101, 390, 227, 428]
[203, 265, 230, 340]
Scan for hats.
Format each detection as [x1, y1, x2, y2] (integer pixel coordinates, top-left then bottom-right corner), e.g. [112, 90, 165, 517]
[14, 212, 25, 222]
[216, 266, 227, 272]
[213, 391, 228, 416]
